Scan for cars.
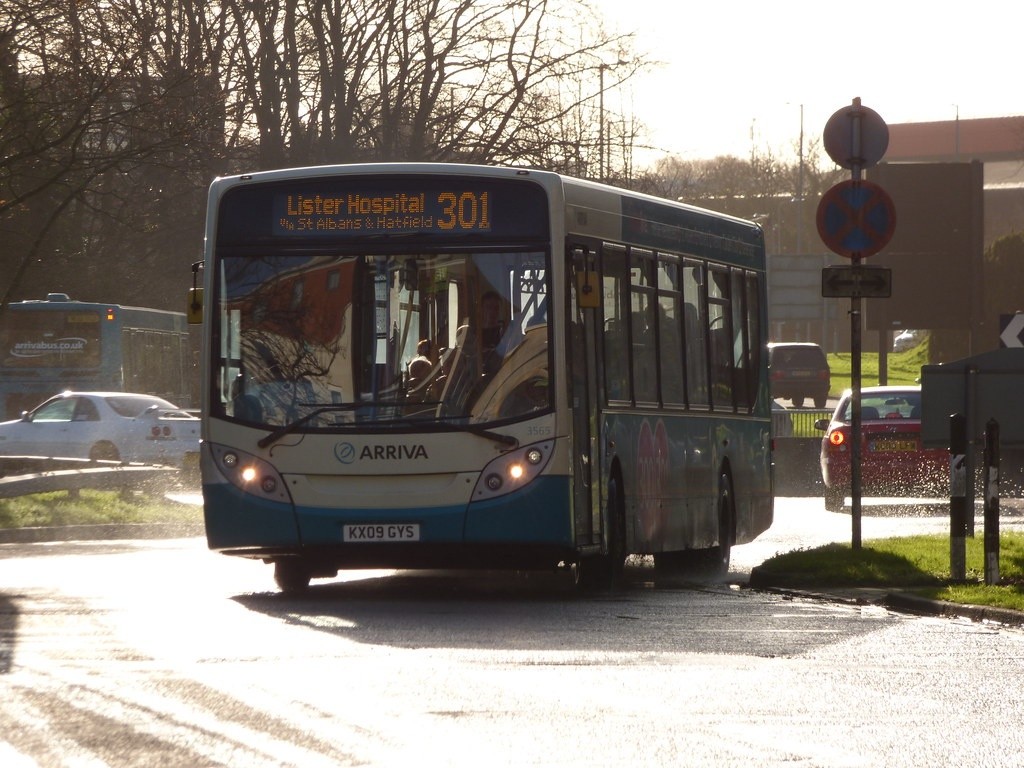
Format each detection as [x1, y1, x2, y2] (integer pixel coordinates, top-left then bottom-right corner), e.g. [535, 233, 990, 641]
[0, 390, 200, 464]
[814, 387, 953, 514]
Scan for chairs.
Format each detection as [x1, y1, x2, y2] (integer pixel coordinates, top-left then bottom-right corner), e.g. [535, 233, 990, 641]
[428, 353, 467, 402]
[417, 340, 441, 367]
[526, 316, 545, 328]
[710, 317, 728, 368]
[405, 358, 433, 401]
[455, 324, 470, 349]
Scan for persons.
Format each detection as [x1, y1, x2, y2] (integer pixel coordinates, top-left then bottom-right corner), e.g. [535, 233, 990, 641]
[480, 293, 503, 328]
[407, 339, 434, 388]
[424, 348, 472, 400]
[455, 324, 474, 346]
[620, 301, 700, 339]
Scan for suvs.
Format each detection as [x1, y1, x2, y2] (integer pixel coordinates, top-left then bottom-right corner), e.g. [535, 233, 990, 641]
[764, 341, 832, 410]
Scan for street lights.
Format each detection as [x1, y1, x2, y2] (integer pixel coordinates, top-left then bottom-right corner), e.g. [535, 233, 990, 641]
[600, 60, 632, 182]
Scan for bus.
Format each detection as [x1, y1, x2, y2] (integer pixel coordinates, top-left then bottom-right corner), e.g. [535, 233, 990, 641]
[185, 161, 774, 601]
[0, 293, 194, 420]
